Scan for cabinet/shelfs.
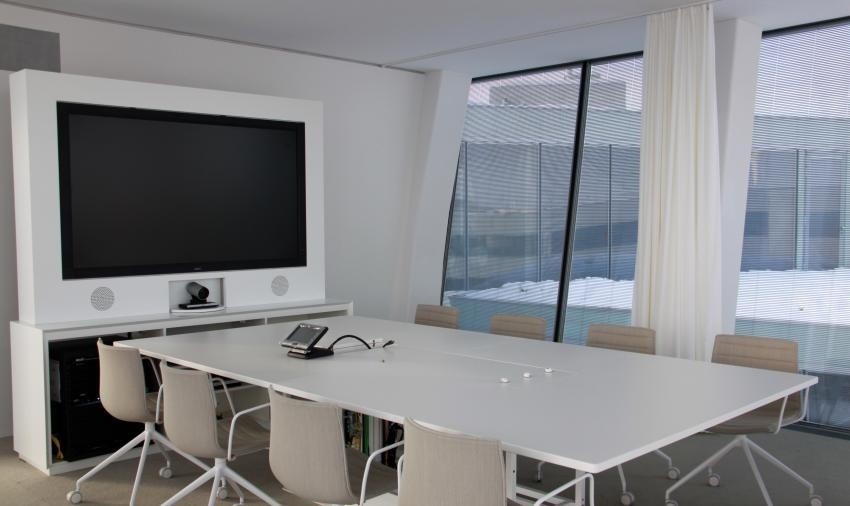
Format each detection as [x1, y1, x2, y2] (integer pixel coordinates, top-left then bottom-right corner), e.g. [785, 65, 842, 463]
[8, 301, 355, 477]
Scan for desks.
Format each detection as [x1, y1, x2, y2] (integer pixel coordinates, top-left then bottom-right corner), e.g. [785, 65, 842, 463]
[111, 314, 821, 506]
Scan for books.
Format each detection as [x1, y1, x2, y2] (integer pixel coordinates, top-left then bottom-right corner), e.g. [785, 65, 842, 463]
[343, 409, 404, 471]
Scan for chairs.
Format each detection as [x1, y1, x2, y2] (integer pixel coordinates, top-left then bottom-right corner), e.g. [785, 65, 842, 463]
[664, 334, 825, 506]
[489, 314, 547, 341]
[358, 414, 506, 506]
[65, 337, 230, 506]
[157, 356, 281, 506]
[413, 303, 459, 330]
[268, 384, 399, 506]
[536, 321, 680, 506]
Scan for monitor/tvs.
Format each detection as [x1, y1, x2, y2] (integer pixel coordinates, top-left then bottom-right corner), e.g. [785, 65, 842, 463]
[10, 68, 326, 325]
